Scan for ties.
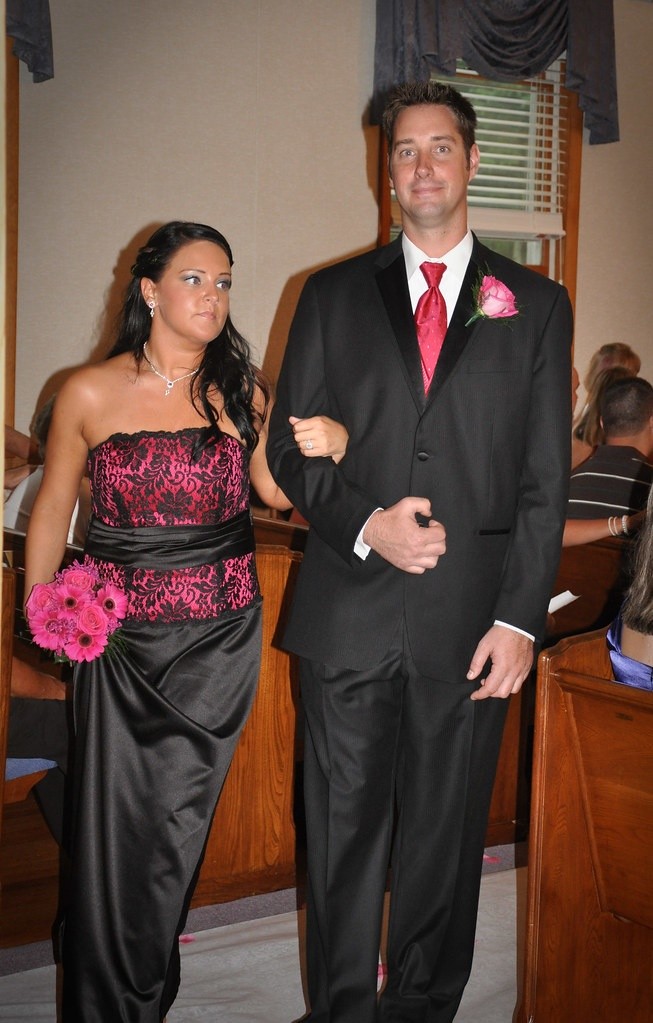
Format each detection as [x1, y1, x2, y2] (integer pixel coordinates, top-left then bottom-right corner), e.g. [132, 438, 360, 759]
[414, 260, 447, 397]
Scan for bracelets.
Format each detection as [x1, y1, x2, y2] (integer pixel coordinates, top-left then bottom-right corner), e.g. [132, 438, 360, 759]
[608, 517, 616, 536]
[613, 517, 621, 535]
[622, 515, 630, 536]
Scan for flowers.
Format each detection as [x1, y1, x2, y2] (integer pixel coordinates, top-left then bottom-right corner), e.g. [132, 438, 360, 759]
[465, 259, 530, 332]
[23, 551, 132, 665]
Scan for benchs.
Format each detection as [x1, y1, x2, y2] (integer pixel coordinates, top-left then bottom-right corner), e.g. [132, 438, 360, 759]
[0, 481, 653, 1023]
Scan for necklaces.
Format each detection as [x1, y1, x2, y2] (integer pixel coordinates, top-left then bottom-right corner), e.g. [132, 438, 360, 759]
[143, 341, 199, 396]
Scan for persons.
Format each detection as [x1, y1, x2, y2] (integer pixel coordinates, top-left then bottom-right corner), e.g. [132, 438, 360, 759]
[4, 391, 91, 547]
[265, 82, 574, 1023]
[561, 343, 653, 691]
[23, 221, 348, 1023]
[6, 655, 74, 847]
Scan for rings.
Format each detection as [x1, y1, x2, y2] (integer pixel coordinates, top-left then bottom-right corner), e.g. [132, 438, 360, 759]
[306, 440, 313, 450]
